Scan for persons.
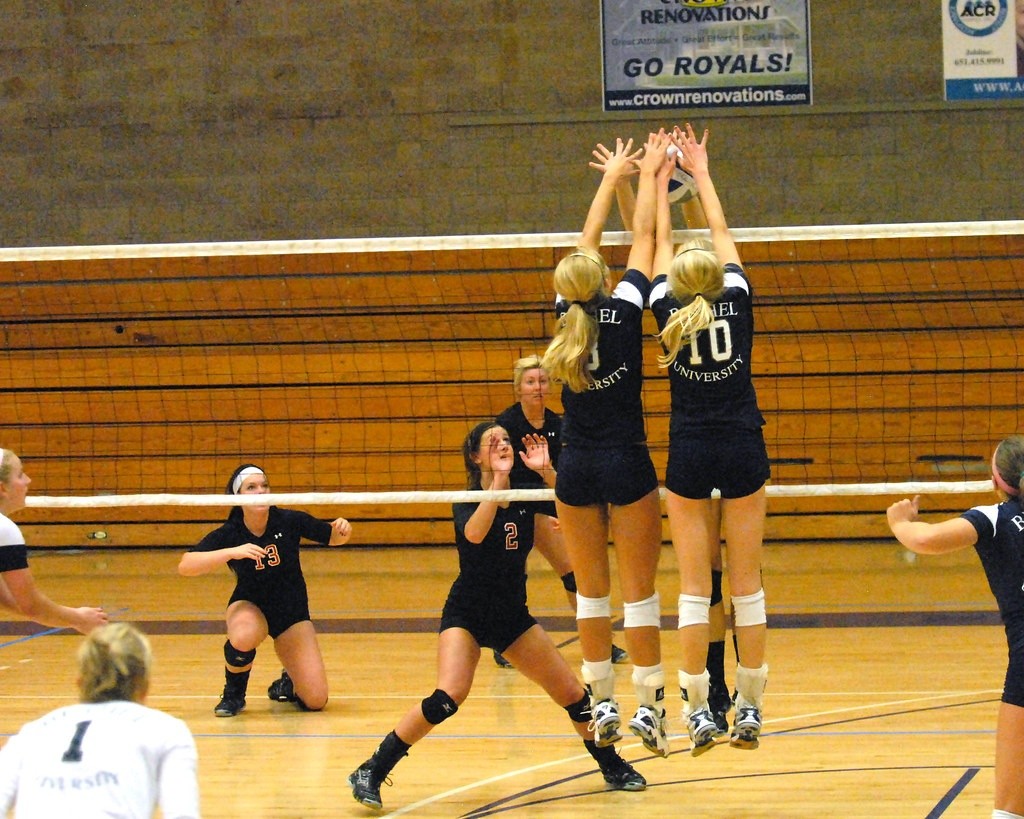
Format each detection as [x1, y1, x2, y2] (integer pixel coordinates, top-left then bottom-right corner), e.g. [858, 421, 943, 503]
[178, 464, 353, 716]
[494, 354, 629, 669]
[0, 449, 109, 634]
[589, 131, 752, 734]
[886, 436, 1024, 819]
[0, 623, 201, 819]
[539, 128, 670, 759]
[346, 422, 646, 810]
[644, 123, 771, 757]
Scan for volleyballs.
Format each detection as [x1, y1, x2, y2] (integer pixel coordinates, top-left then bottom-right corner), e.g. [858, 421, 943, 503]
[667, 142, 709, 204]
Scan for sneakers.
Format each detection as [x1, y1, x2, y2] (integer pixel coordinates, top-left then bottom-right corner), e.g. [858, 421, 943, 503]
[579, 698, 623, 748]
[628, 704, 670, 759]
[601, 758, 646, 791]
[729, 705, 763, 750]
[347, 760, 394, 809]
[686, 707, 717, 758]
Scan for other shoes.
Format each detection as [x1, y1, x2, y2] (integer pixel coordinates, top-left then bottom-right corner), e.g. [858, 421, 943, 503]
[711, 710, 729, 733]
[214, 685, 246, 717]
[613, 644, 629, 663]
[494, 650, 513, 669]
[267, 667, 294, 703]
[731, 687, 738, 705]
